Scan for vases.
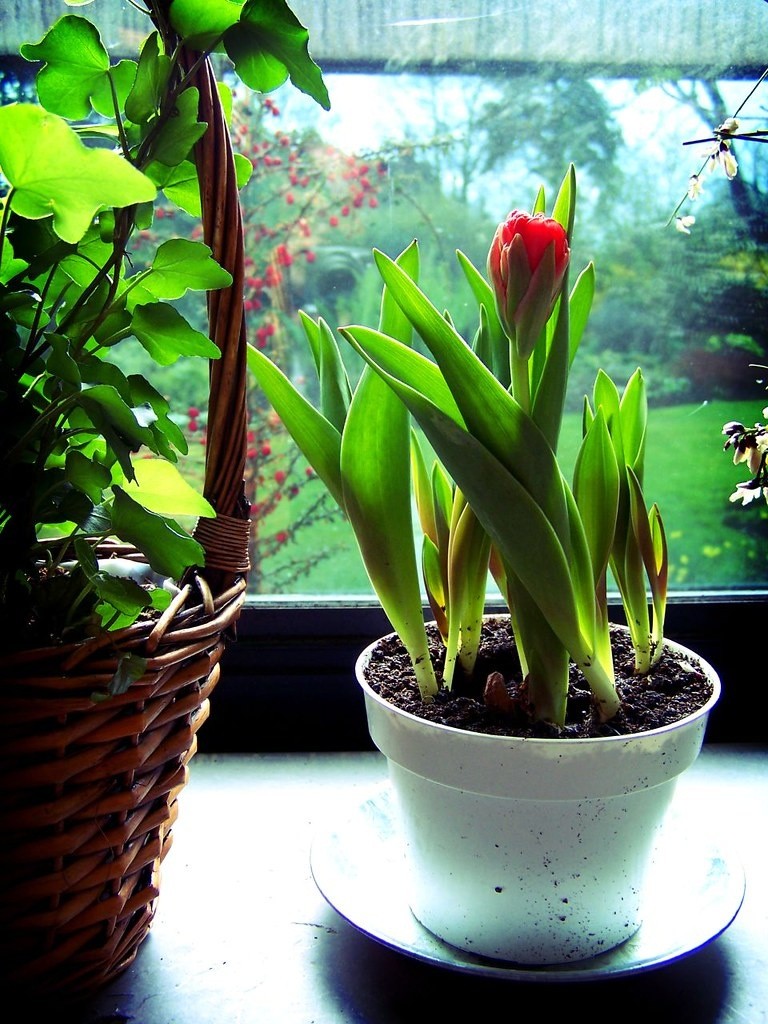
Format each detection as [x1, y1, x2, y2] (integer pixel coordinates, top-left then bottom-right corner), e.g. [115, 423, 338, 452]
[353, 614, 722, 969]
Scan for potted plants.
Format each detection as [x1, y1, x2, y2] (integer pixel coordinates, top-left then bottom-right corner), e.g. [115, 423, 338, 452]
[0, 0, 330, 1024]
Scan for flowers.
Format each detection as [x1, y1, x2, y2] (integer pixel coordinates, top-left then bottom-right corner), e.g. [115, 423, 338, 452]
[246, 161, 668, 729]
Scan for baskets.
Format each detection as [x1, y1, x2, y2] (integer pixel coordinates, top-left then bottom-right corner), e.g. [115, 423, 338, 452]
[0, 0, 251, 1008]
